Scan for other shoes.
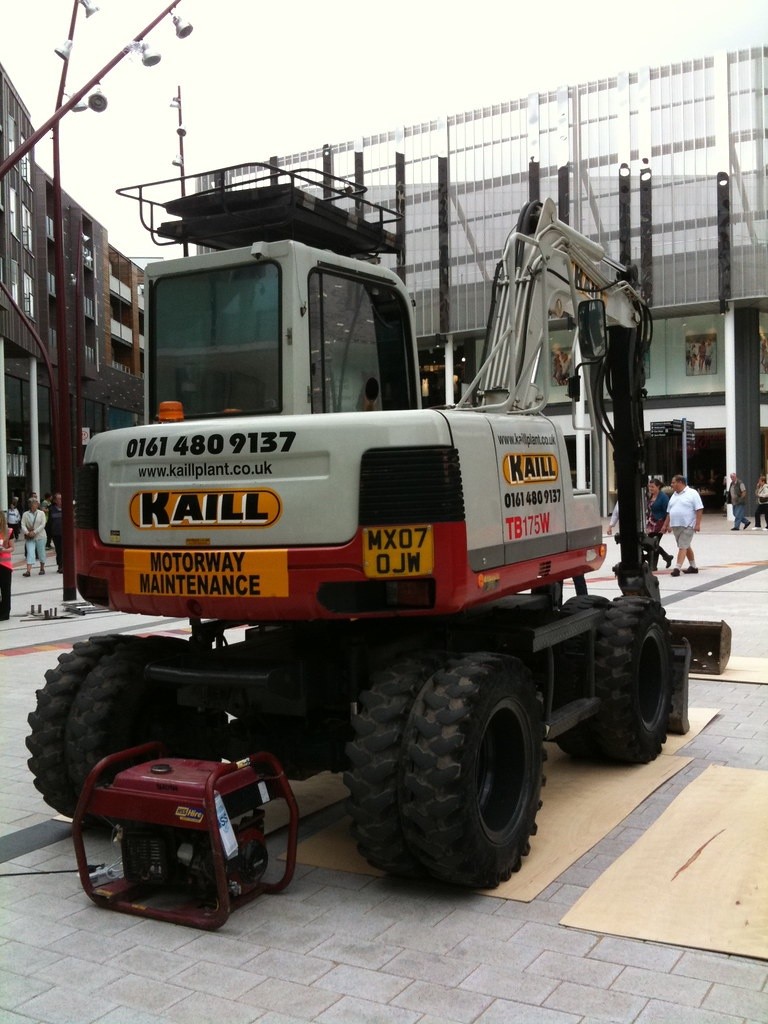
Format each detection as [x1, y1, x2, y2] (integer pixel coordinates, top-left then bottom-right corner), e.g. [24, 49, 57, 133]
[683, 567, 698, 574]
[23, 571, 30, 576]
[39, 570, 45, 575]
[731, 527, 739, 530]
[762, 527, 768, 531]
[744, 521, 751, 529]
[650, 565, 657, 572]
[751, 526, 761, 530]
[58, 566, 63, 573]
[664, 554, 674, 568]
[45, 546, 54, 550]
[672, 568, 682, 577]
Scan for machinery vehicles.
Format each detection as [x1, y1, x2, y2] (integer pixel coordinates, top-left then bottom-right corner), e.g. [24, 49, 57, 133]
[26, 150, 731, 891]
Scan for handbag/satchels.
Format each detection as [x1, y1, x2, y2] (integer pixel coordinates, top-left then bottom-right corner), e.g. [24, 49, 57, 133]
[758, 486, 768, 502]
[25, 528, 35, 539]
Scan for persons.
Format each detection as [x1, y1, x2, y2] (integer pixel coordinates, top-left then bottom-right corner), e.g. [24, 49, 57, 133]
[8, 496, 22, 540]
[644, 479, 674, 571]
[21, 497, 48, 577]
[606, 499, 619, 536]
[0, 510, 15, 622]
[24, 493, 64, 574]
[729, 473, 751, 531]
[659, 475, 704, 577]
[760, 339, 768, 375]
[752, 475, 768, 531]
[552, 352, 573, 386]
[686, 339, 712, 375]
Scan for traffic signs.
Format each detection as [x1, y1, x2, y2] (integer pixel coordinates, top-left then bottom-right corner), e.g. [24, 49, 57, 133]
[649, 419, 697, 445]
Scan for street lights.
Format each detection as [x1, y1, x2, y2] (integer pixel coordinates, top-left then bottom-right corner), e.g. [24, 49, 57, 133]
[170, 93, 188, 260]
[54, 0, 101, 600]
